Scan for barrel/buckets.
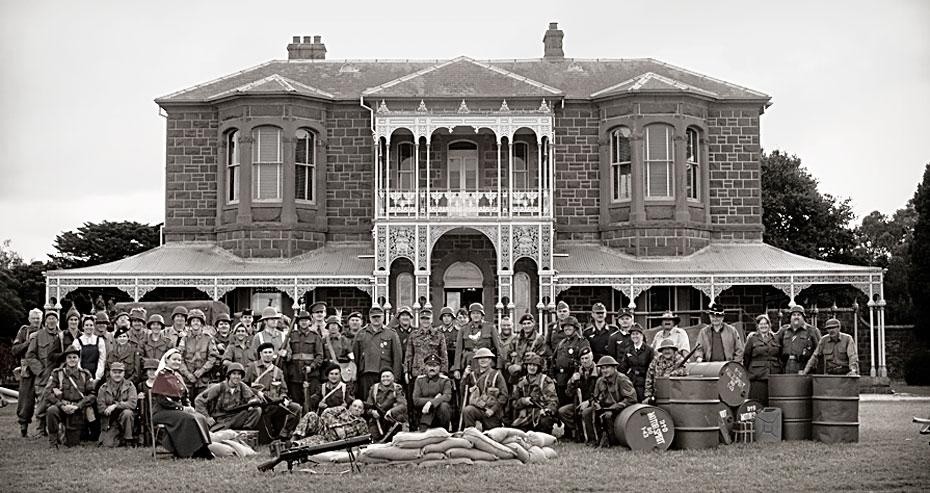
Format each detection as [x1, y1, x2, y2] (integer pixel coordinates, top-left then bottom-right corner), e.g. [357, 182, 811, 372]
[812, 375, 860, 443]
[614, 403, 675, 453]
[737, 399, 764, 439]
[769, 375, 811, 440]
[753, 405, 784, 442]
[684, 360, 751, 406]
[655, 376, 720, 450]
[717, 403, 733, 435]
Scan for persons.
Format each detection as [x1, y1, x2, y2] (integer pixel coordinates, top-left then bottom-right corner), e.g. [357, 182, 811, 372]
[11, 300, 857, 459]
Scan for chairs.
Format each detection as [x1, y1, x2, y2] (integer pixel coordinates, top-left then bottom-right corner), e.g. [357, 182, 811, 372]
[148, 388, 175, 463]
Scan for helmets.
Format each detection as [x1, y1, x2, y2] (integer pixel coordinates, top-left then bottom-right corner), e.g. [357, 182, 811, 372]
[657, 339, 679, 351]
[523, 352, 544, 368]
[595, 355, 620, 366]
[788, 304, 807, 318]
[148, 305, 233, 326]
[472, 347, 496, 359]
[225, 362, 246, 379]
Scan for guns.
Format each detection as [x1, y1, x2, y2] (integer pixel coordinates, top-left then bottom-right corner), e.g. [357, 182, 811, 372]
[256, 434, 373, 473]
[209, 398, 285, 430]
[363, 396, 396, 421]
[275, 318, 297, 368]
[531, 397, 551, 416]
[671, 343, 700, 373]
[304, 366, 310, 414]
[573, 365, 586, 443]
[407, 362, 418, 432]
[264, 395, 296, 416]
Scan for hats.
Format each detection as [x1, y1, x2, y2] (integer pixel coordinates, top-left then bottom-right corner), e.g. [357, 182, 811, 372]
[424, 353, 443, 365]
[45, 300, 147, 338]
[825, 318, 841, 329]
[661, 309, 675, 319]
[232, 301, 343, 352]
[557, 300, 645, 357]
[346, 302, 486, 319]
[325, 362, 342, 377]
[518, 313, 536, 324]
[64, 344, 83, 357]
[109, 361, 126, 371]
[144, 358, 159, 371]
[379, 367, 395, 376]
[708, 304, 725, 315]
